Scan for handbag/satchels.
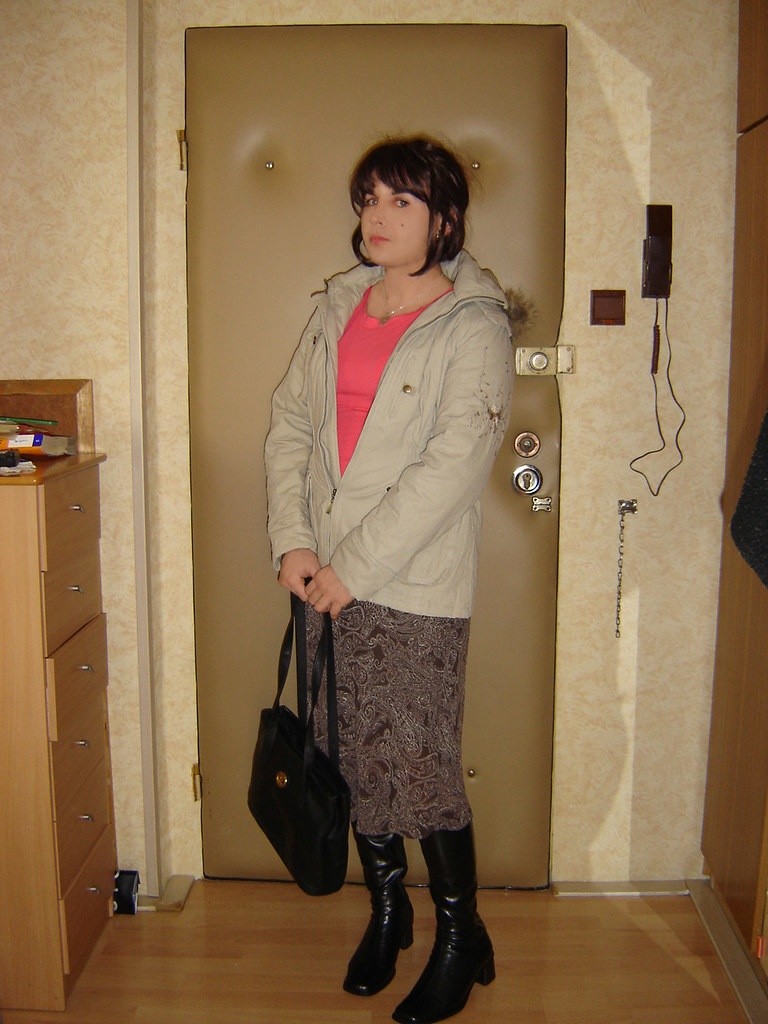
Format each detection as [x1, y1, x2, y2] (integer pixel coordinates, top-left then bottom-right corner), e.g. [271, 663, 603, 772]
[247, 580, 349, 897]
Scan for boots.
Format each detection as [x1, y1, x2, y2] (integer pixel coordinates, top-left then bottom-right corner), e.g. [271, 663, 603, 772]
[391, 820, 495, 1024]
[343, 821, 414, 998]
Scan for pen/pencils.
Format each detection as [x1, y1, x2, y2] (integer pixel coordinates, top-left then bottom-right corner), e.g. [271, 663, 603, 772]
[0, 416, 58, 426]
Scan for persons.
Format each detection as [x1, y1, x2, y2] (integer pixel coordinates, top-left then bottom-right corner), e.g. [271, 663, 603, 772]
[264, 132, 515, 1024]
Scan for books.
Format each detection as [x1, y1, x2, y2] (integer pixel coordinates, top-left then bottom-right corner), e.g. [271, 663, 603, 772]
[0, 419, 77, 456]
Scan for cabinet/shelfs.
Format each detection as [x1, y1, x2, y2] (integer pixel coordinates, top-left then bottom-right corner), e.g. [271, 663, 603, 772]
[0, 380, 116, 1012]
[688, 0, 768, 1024]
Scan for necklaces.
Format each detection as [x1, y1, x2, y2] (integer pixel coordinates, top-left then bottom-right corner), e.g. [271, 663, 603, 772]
[379, 272, 445, 325]
